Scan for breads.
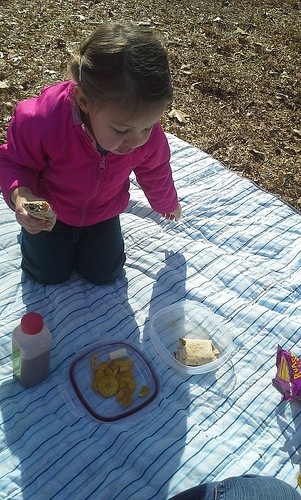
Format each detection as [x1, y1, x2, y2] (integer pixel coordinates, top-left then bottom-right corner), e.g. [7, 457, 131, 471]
[90, 353, 148, 406]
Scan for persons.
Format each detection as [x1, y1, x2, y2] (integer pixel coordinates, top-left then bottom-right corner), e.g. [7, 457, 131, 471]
[0, 23, 181, 287]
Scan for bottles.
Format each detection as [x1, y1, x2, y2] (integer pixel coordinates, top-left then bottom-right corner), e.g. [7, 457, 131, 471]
[12, 312, 51, 387]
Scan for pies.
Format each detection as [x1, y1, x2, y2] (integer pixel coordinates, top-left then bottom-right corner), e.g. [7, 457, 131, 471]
[174, 338, 220, 366]
[22, 201, 56, 232]
